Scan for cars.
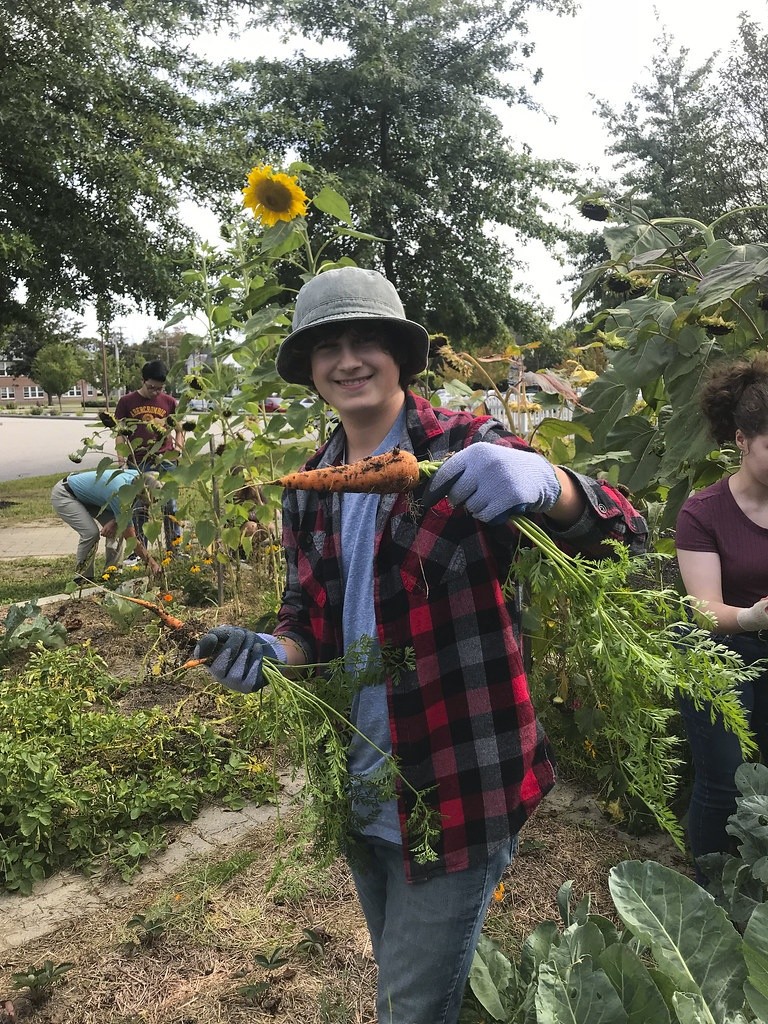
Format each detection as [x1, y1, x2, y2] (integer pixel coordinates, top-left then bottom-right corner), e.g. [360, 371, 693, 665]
[187, 396, 331, 414]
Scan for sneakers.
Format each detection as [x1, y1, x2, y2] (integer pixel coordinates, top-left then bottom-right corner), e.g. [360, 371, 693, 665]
[122, 553, 141, 567]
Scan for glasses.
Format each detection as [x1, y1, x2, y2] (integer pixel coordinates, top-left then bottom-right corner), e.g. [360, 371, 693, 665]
[144, 380, 163, 394]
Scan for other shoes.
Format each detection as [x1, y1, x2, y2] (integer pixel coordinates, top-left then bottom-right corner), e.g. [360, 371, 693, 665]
[98, 575, 109, 583]
[74, 577, 97, 586]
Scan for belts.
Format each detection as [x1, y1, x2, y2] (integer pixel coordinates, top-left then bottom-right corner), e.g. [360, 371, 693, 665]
[62, 477, 77, 499]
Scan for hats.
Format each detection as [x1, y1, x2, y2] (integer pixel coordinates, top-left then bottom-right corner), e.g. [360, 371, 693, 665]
[131, 471, 165, 503]
[275, 267, 430, 385]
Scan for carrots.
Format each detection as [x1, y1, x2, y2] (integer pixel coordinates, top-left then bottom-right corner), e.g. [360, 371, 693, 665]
[183, 657, 207, 668]
[75, 573, 206, 640]
[224, 449, 420, 497]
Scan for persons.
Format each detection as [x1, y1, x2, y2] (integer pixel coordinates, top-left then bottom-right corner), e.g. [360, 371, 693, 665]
[219, 466, 281, 562]
[674, 350, 768, 893]
[194, 265, 647, 1024]
[52, 469, 163, 584]
[115, 360, 190, 566]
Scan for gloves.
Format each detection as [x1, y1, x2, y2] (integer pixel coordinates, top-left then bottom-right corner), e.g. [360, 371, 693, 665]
[421, 441, 561, 527]
[193, 625, 288, 694]
